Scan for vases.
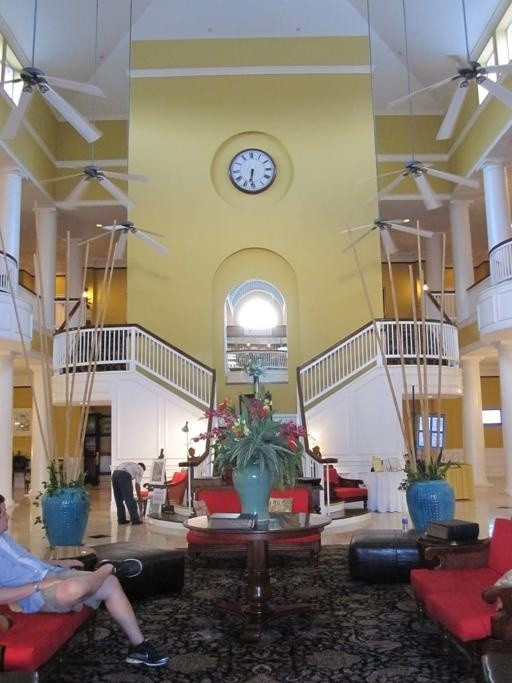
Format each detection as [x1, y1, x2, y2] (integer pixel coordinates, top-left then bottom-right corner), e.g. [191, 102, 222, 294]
[232, 457, 278, 521]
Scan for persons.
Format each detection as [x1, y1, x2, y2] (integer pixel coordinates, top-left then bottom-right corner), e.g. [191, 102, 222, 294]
[111, 461, 147, 525]
[0, 493, 170, 666]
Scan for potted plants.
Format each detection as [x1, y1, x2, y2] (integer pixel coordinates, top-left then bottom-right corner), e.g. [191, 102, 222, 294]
[13, 450, 30, 489]
[32, 459, 88, 545]
[397, 449, 461, 526]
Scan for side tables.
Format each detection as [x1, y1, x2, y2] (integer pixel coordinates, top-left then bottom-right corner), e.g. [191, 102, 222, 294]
[417, 532, 479, 568]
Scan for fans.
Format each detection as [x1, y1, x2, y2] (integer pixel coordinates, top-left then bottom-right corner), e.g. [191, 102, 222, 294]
[77, 221, 167, 260]
[338, 220, 431, 253]
[0, 1, 104, 143]
[35, 0, 145, 211]
[358, 0, 477, 210]
[386, 0, 510, 140]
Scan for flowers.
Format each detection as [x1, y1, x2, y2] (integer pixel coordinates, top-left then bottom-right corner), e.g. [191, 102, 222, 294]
[191, 392, 307, 488]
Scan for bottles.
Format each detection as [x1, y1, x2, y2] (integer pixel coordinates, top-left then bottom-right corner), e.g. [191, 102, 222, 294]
[402, 516, 408, 531]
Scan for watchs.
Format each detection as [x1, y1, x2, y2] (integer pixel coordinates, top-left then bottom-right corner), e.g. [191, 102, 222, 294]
[34, 580, 42, 593]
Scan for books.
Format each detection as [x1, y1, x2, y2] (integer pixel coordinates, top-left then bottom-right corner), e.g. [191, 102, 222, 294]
[426, 519, 479, 540]
[208, 511, 258, 528]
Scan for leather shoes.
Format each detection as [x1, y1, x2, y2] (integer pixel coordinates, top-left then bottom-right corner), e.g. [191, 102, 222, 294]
[133, 521, 143, 524]
[118, 520, 130, 523]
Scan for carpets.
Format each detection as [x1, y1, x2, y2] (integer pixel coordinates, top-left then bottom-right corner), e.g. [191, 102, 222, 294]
[40, 542, 488, 683]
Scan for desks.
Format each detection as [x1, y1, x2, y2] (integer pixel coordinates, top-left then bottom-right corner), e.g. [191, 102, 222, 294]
[439, 463, 473, 502]
[182, 510, 331, 643]
[358, 470, 407, 512]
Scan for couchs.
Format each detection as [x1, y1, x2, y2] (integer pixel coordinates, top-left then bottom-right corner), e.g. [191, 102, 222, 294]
[410, 518, 511, 677]
[186, 486, 322, 567]
[0, 604, 95, 683]
[139, 472, 187, 504]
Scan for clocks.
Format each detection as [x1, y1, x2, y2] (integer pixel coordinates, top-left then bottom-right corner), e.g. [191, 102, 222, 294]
[227, 148, 274, 194]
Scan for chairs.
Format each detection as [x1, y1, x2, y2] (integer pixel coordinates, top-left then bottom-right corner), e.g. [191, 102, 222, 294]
[314, 445, 368, 509]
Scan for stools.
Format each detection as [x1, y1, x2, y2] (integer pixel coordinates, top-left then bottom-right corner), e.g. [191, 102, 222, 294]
[347, 528, 418, 579]
[91, 542, 181, 600]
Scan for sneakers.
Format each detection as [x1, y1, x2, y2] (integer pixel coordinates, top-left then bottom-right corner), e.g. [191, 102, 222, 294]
[126, 639, 170, 666]
[100, 558, 142, 577]
